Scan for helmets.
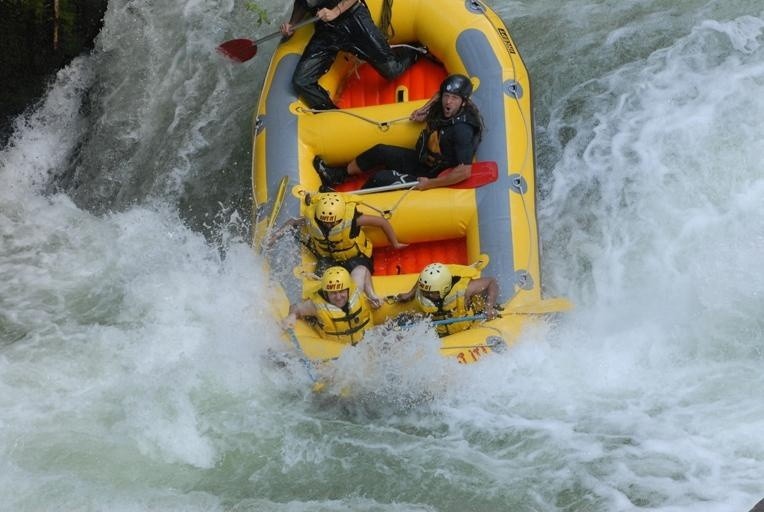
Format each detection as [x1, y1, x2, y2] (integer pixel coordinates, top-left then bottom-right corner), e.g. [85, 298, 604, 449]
[440, 74, 472, 97]
[315, 193, 345, 223]
[418, 262, 452, 299]
[321, 266, 351, 299]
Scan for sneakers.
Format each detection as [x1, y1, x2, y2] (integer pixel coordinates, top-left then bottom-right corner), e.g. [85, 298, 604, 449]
[413, 43, 434, 60]
[314, 155, 338, 186]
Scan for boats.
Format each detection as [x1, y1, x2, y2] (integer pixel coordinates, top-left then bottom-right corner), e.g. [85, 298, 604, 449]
[254, 0, 550, 409]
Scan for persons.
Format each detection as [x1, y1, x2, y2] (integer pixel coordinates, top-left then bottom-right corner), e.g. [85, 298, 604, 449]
[280, 0, 429, 110]
[289, 265, 383, 347]
[394, 254, 499, 339]
[269, 192, 409, 274]
[313, 74, 485, 191]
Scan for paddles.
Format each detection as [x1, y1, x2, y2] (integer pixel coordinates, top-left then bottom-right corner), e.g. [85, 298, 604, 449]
[258, 176, 291, 257]
[263, 283, 324, 393]
[394, 299, 575, 332]
[216, 17, 324, 63]
[306, 162, 498, 207]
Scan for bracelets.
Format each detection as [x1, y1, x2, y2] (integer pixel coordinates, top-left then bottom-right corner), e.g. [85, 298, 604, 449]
[337, 5, 341, 15]
